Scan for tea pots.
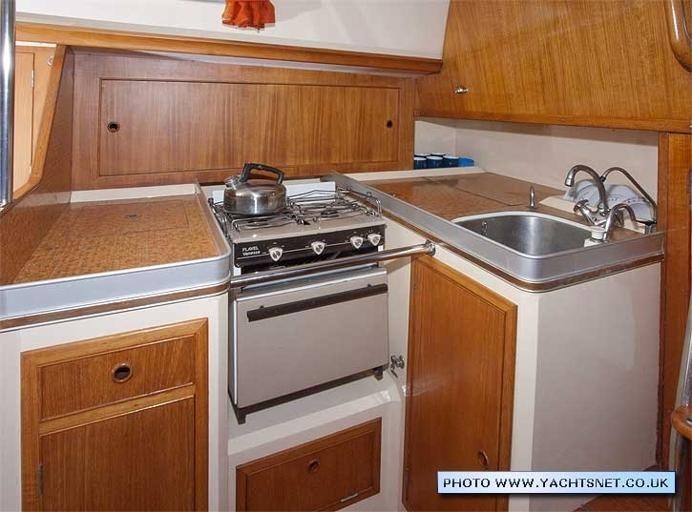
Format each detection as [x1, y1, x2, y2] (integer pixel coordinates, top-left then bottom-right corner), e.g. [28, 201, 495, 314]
[222, 161, 287, 219]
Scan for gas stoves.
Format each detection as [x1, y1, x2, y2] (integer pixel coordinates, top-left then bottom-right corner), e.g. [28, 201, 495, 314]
[207, 193, 388, 268]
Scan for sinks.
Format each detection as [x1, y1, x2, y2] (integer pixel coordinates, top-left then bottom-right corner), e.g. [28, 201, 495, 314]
[456, 215, 592, 258]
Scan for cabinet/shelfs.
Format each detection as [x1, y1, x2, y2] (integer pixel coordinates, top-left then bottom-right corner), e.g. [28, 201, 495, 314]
[382, 234, 672, 512]
[219, 385, 399, 512]
[67, 45, 415, 195]
[5, 293, 231, 512]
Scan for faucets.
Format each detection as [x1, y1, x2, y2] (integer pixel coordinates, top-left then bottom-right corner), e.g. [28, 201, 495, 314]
[599, 166, 658, 213]
[564, 164, 610, 215]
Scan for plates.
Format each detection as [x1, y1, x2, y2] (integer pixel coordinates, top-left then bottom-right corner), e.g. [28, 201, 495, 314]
[565, 176, 656, 224]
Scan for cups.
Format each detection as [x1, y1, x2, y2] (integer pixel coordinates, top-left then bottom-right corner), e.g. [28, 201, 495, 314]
[414, 152, 475, 169]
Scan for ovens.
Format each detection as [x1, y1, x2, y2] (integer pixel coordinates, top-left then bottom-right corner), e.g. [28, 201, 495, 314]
[226, 234, 437, 424]
